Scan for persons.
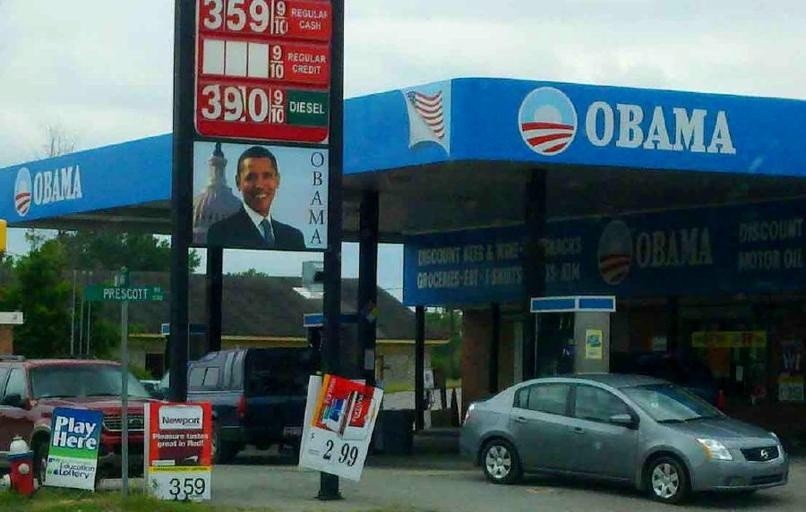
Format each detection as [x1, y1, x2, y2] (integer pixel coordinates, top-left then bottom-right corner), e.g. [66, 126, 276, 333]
[206, 146, 309, 250]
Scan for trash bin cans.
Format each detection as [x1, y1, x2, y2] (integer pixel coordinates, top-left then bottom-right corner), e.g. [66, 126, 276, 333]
[373, 408, 415, 454]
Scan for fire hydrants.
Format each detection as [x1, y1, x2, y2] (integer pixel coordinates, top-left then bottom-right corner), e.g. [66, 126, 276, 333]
[0, 433, 38, 496]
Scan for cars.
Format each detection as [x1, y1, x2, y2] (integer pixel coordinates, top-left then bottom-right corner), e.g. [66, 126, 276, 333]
[456, 373, 791, 503]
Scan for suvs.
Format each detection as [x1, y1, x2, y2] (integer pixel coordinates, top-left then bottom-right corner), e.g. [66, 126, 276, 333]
[156, 345, 326, 462]
[0, 354, 152, 482]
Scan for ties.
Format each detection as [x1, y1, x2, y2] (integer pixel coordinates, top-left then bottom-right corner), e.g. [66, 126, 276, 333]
[259, 219, 274, 248]
